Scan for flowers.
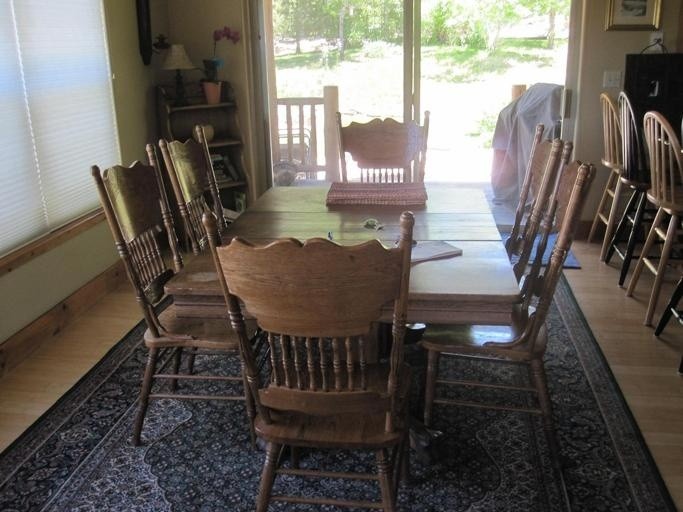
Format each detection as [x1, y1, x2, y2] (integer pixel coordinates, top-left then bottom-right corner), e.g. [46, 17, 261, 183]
[211, 26, 237, 55]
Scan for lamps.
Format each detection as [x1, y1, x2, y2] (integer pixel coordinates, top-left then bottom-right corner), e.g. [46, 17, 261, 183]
[160, 45, 193, 103]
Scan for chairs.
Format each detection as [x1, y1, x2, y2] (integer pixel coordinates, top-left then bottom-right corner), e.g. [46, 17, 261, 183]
[425, 139, 597, 425]
[91, 143, 267, 447]
[200, 210, 415, 512]
[605, 92, 648, 288]
[654, 273, 683, 371]
[504, 122, 561, 285]
[160, 124, 227, 250]
[588, 92, 626, 260]
[626, 111, 681, 326]
[337, 111, 430, 183]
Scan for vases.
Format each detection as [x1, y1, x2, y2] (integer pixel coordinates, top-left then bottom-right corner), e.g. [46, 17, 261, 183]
[202, 57, 222, 74]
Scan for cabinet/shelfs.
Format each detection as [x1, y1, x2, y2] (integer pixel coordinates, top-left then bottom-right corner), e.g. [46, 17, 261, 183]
[155, 82, 254, 242]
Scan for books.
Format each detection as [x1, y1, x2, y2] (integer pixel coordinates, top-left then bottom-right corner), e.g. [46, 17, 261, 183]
[411, 241, 463, 262]
[224, 191, 246, 224]
[211, 154, 238, 182]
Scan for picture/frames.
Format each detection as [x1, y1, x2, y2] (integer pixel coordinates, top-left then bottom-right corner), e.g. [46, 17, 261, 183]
[604, 0, 663, 32]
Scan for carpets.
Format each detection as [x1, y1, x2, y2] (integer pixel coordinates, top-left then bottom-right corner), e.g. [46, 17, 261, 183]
[0, 269, 682, 512]
[500, 232, 581, 268]
